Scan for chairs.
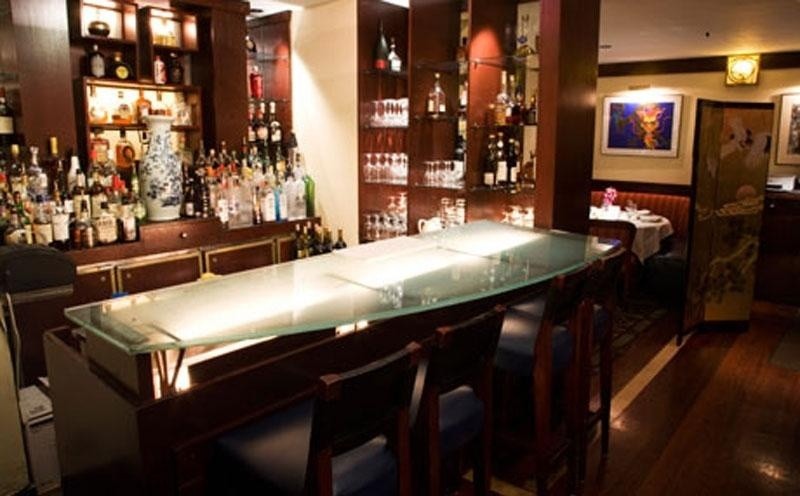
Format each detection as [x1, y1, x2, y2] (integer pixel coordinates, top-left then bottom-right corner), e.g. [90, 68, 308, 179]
[488, 301, 588, 495]
[216, 341, 420, 496]
[579, 223, 634, 454]
[411, 306, 507, 494]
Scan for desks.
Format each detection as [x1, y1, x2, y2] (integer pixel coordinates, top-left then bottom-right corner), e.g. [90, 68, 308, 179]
[42, 223, 624, 496]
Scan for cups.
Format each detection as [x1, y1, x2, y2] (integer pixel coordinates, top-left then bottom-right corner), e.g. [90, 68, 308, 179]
[439, 197, 466, 229]
[608, 205, 621, 219]
[625, 203, 637, 221]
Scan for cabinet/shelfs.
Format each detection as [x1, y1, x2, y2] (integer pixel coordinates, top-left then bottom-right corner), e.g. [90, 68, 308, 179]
[203, 217, 316, 276]
[246, 10, 292, 178]
[11, 0, 250, 176]
[356, 1, 600, 244]
[16, 248, 207, 387]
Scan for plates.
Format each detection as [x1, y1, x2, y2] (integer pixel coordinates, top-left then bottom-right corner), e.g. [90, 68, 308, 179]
[636, 209, 651, 218]
[639, 215, 661, 221]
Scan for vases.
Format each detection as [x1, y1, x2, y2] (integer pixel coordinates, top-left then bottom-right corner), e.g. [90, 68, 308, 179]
[139, 115, 184, 222]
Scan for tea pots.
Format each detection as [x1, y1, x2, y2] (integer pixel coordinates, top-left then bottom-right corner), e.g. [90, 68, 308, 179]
[418, 218, 443, 234]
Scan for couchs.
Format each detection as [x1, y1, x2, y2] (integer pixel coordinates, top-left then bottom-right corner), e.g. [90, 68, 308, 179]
[590, 176, 695, 345]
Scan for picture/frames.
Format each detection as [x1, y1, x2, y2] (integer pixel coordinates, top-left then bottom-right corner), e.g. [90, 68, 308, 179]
[775, 93, 800, 167]
[600, 93, 683, 158]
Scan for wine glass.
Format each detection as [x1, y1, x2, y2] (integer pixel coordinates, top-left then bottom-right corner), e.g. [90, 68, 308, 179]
[363, 153, 408, 185]
[369, 97, 410, 127]
[421, 160, 454, 186]
[362, 192, 407, 240]
[500, 204, 535, 228]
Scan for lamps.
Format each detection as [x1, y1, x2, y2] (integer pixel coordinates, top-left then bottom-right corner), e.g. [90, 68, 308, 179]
[725, 54, 760, 86]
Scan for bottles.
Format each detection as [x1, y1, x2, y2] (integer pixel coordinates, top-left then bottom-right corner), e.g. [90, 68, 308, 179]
[454, 134, 464, 182]
[0, 86, 17, 165]
[85, 84, 193, 126]
[180, 101, 347, 261]
[427, 71, 537, 126]
[89, 11, 109, 37]
[0, 126, 148, 251]
[82, 42, 184, 85]
[483, 132, 524, 188]
[250, 64, 263, 100]
[370, 17, 401, 72]
